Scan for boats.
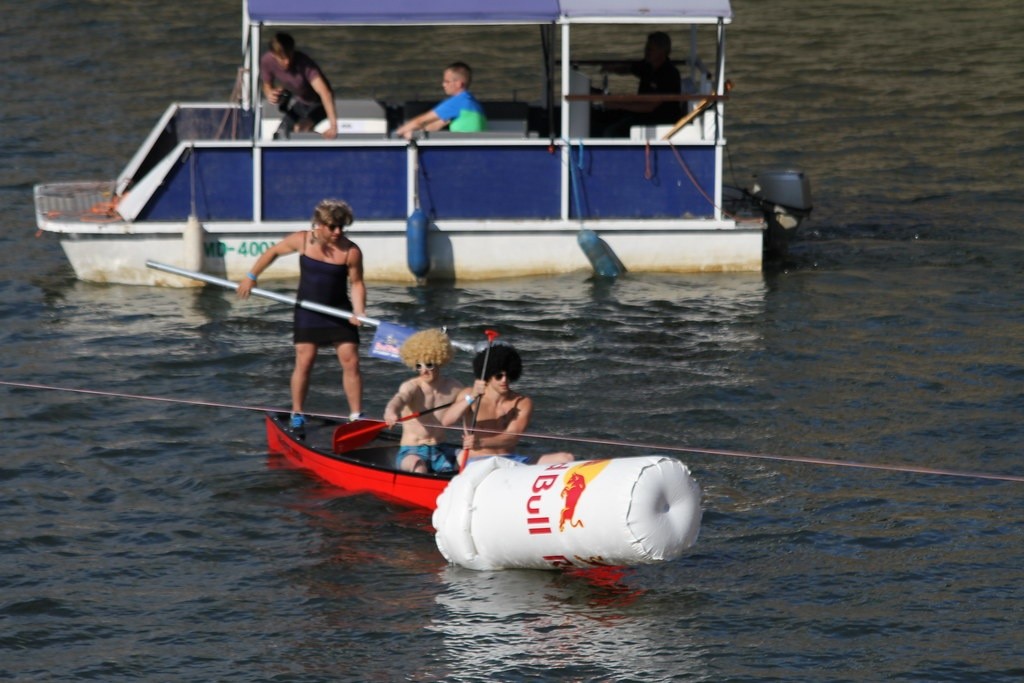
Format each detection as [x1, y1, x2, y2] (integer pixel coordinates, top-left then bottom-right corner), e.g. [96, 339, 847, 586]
[29, 0, 814, 288]
[265, 407, 453, 511]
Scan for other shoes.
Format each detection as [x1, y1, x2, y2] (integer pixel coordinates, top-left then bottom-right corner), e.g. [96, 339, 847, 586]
[346, 412, 363, 424]
[290, 412, 304, 428]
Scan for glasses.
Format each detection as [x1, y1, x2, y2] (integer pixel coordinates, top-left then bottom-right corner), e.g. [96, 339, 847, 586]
[324, 222, 342, 231]
[494, 372, 513, 381]
[415, 363, 434, 370]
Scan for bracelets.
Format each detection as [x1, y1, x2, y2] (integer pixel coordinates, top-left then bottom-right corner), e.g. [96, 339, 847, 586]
[248, 273, 256, 280]
[465, 396, 474, 403]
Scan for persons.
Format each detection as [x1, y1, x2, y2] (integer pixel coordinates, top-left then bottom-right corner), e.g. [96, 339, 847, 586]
[384, 328, 464, 474]
[632, 33, 681, 118]
[443, 344, 574, 500]
[394, 62, 487, 134]
[239, 197, 368, 440]
[260, 32, 338, 138]
